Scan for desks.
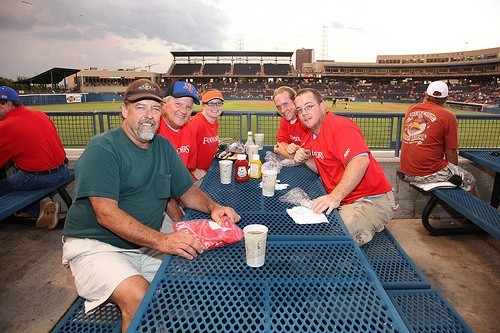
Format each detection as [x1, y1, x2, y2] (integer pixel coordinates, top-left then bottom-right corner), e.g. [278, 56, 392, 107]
[127, 238, 410, 333]
[459, 148, 500, 209]
[184, 144, 356, 240]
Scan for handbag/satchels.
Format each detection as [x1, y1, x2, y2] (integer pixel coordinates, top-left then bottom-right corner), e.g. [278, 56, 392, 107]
[171, 216, 244, 252]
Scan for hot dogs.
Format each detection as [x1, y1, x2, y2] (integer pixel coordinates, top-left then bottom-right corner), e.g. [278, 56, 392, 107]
[219, 151, 232, 159]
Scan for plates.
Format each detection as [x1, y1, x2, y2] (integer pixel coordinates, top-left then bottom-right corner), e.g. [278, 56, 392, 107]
[217, 154, 236, 160]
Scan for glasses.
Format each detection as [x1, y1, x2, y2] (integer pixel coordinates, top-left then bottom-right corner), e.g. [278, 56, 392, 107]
[294, 102, 321, 116]
[203, 102, 224, 107]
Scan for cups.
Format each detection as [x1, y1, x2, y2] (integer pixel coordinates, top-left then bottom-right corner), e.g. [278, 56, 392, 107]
[255, 134, 264, 150]
[219, 160, 233, 185]
[249, 145, 258, 162]
[262, 170, 277, 196]
[243, 224, 268, 267]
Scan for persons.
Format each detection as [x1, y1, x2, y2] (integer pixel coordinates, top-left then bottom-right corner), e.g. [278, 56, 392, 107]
[190, 90, 226, 181]
[156, 81, 200, 233]
[198, 79, 312, 98]
[447, 79, 500, 104]
[0, 86, 68, 229]
[323, 81, 427, 110]
[62, 79, 241, 333]
[400, 81, 477, 196]
[293, 89, 400, 246]
[274, 86, 311, 160]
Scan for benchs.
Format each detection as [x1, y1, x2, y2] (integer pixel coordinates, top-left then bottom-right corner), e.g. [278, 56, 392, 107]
[360, 227, 472, 333]
[0, 169, 74, 227]
[397, 173, 500, 241]
[50, 295, 122, 333]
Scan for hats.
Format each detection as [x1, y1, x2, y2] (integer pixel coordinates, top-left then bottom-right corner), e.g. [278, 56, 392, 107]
[202, 89, 225, 105]
[164, 80, 201, 106]
[122, 79, 167, 104]
[0, 86, 19, 100]
[427, 81, 449, 98]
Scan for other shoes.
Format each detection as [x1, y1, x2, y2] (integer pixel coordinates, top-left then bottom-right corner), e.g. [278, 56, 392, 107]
[35, 197, 61, 229]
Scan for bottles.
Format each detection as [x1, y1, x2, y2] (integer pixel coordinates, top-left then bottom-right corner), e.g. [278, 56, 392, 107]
[235, 154, 248, 181]
[250, 155, 261, 179]
[245, 132, 254, 154]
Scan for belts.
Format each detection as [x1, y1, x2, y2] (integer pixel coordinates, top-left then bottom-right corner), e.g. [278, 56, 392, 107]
[24, 157, 69, 175]
